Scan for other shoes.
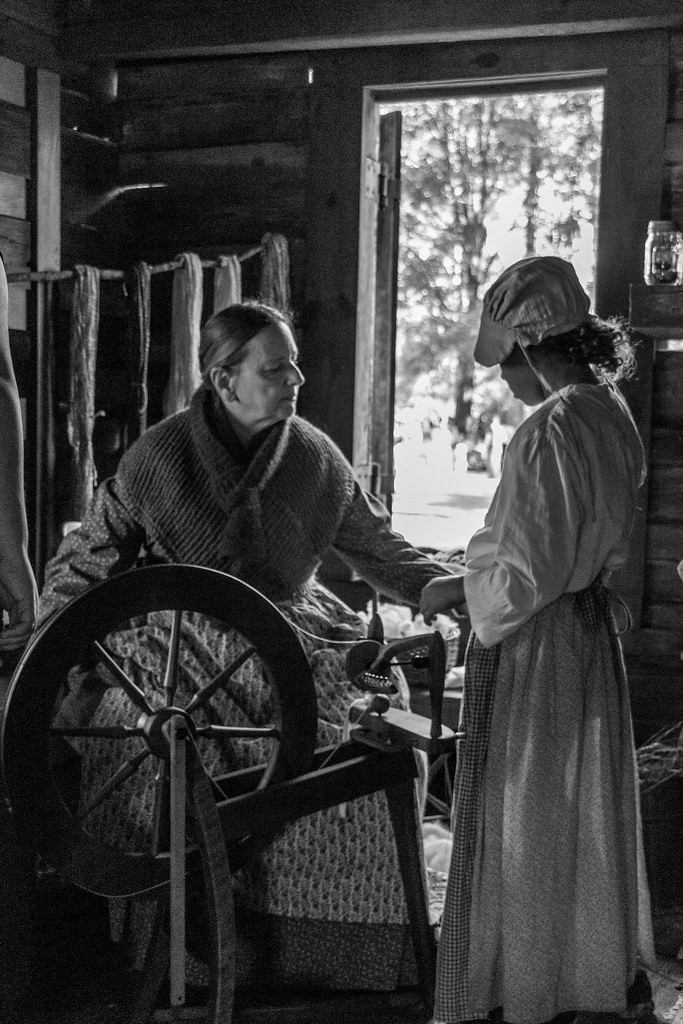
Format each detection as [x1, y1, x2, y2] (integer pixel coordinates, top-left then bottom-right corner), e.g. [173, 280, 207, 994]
[617, 972, 654, 1019]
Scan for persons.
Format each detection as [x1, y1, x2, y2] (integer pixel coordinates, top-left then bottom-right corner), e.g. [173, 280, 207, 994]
[0, 251, 39, 652]
[422, 258, 658, 1023]
[49, 301, 473, 1003]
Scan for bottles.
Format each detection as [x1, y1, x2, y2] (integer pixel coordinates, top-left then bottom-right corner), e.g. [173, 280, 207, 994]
[644, 220, 683, 288]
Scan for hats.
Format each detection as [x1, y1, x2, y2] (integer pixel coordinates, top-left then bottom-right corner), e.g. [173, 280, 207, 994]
[475, 257, 591, 368]
